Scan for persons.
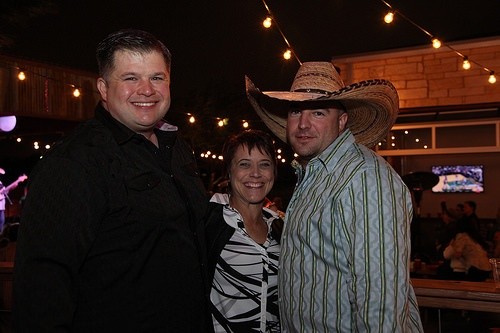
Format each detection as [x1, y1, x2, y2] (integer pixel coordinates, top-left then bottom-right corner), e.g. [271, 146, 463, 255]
[203, 128, 284, 333]
[9, 29, 211, 333]
[436, 201, 500, 282]
[0, 168, 18, 234]
[244, 61, 424, 333]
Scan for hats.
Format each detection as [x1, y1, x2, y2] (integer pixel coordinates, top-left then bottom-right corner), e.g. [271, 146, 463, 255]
[245, 62, 400, 148]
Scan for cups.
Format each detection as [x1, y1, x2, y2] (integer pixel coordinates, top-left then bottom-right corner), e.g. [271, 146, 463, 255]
[489, 257, 500, 288]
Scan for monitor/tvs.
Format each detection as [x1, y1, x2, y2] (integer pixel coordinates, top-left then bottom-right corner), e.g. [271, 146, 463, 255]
[432, 163, 486, 193]
